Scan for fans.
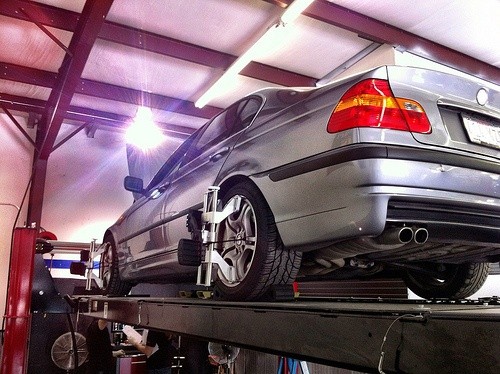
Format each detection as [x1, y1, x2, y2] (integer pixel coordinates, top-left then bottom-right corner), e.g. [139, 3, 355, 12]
[207, 342, 240, 374]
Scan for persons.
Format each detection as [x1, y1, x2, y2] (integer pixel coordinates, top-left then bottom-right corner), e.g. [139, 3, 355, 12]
[86, 317, 127, 374]
[128, 330, 173, 374]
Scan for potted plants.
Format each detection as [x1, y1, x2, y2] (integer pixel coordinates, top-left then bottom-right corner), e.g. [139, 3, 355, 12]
[113, 337, 123, 345]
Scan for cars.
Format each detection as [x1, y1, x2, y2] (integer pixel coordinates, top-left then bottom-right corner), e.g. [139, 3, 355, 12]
[99, 65, 500, 300]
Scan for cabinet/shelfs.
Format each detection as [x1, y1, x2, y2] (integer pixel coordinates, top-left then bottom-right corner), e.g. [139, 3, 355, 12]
[115, 355, 148, 374]
